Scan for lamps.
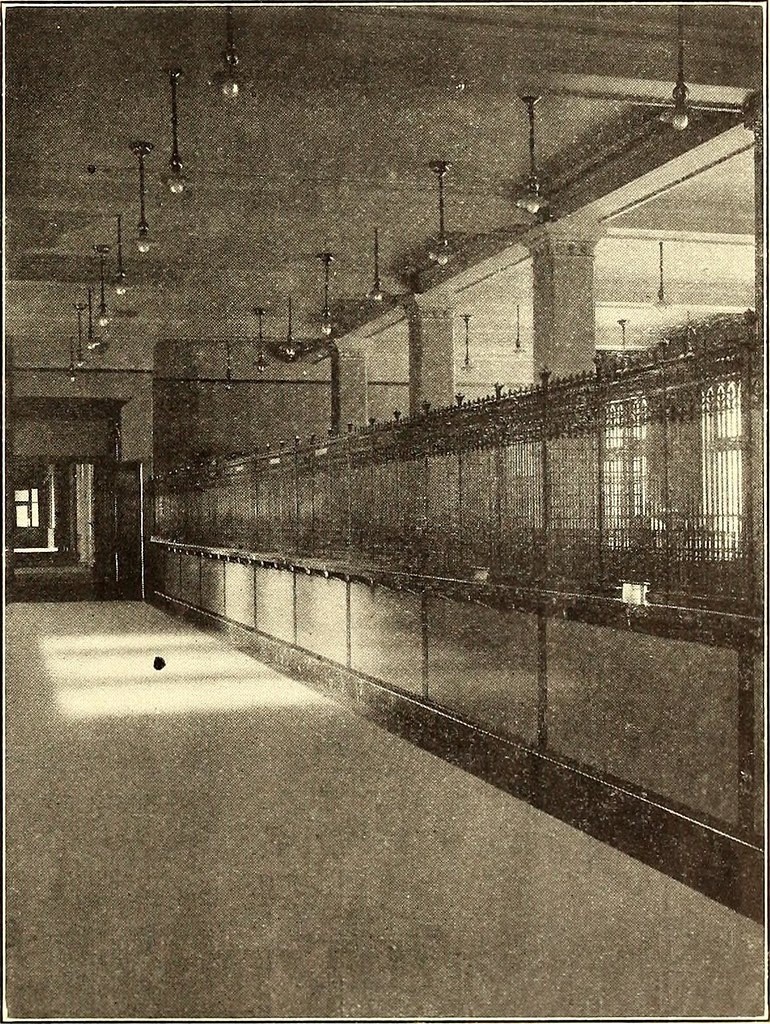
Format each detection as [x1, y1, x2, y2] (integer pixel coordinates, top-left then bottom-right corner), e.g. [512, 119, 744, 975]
[129, 139, 160, 255]
[316, 250, 336, 337]
[657, 5, 703, 130]
[253, 307, 270, 373]
[107, 213, 132, 296]
[511, 91, 551, 216]
[366, 225, 389, 306]
[72, 302, 90, 368]
[159, 66, 196, 196]
[276, 289, 302, 366]
[221, 342, 238, 391]
[66, 340, 78, 383]
[94, 242, 114, 329]
[83, 281, 102, 354]
[423, 159, 458, 267]
[204, 8, 254, 97]
